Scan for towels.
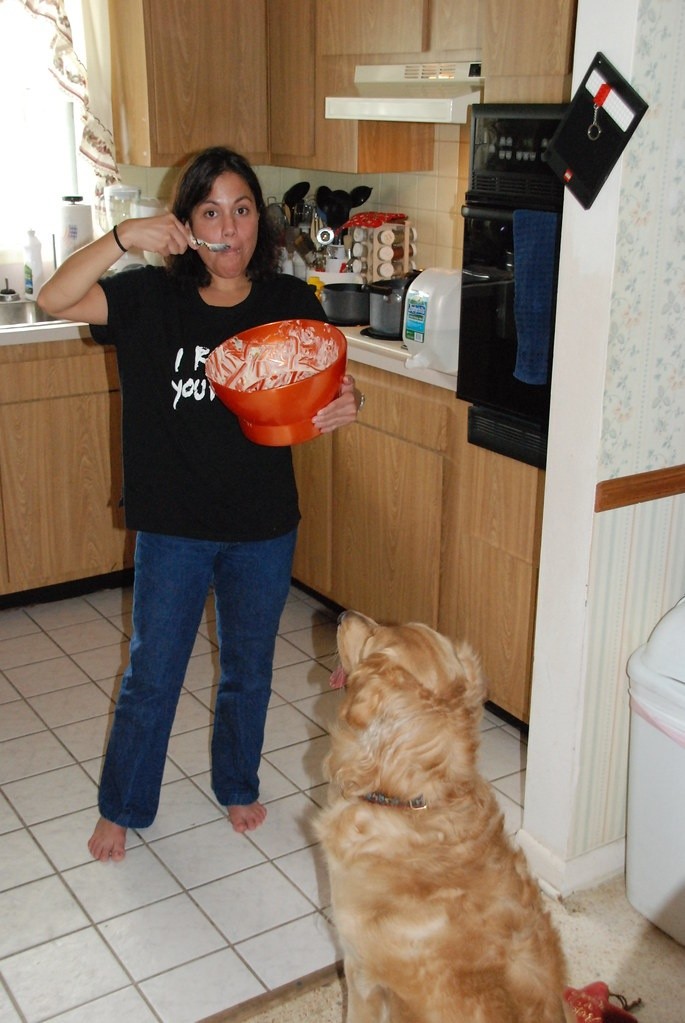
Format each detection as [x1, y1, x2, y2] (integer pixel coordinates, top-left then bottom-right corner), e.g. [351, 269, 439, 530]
[512, 208, 558, 385]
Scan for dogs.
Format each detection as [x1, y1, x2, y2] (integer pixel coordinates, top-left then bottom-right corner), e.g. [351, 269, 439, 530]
[312, 607, 577, 1023]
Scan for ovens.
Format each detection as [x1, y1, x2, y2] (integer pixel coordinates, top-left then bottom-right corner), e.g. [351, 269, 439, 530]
[455, 103, 570, 473]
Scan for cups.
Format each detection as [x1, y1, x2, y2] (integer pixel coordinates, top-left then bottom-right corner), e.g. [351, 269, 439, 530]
[353, 260, 368, 274]
[352, 243, 368, 257]
[379, 227, 417, 277]
[353, 228, 367, 242]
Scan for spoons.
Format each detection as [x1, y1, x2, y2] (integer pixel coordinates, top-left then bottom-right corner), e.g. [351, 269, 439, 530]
[191, 237, 230, 252]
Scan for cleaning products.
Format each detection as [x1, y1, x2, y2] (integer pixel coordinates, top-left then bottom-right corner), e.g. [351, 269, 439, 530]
[23, 228, 44, 300]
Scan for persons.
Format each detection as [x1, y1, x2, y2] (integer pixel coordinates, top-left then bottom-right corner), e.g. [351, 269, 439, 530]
[37, 147, 362, 863]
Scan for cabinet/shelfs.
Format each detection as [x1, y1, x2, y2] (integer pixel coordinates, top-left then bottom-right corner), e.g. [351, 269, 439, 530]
[109, 0, 575, 176]
[286, 358, 550, 726]
[0, 339, 136, 597]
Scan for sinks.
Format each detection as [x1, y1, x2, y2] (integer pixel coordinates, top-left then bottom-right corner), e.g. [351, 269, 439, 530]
[0, 298, 63, 328]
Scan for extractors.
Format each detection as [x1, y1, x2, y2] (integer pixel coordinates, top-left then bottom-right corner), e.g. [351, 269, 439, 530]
[324, 62, 484, 123]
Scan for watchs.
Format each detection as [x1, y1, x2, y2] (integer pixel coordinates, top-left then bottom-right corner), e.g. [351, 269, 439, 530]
[361, 393, 365, 406]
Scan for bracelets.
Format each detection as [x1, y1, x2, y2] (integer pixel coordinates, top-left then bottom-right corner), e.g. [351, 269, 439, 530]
[113, 224, 128, 253]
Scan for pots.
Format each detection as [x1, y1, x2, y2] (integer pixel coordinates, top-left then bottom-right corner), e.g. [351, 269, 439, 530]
[370, 278, 412, 334]
[322, 283, 369, 325]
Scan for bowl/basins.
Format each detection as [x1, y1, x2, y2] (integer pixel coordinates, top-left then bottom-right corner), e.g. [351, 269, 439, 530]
[130, 199, 168, 266]
[205, 320, 348, 446]
[104, 186, 141, 230]
[327, 245, 344, 259]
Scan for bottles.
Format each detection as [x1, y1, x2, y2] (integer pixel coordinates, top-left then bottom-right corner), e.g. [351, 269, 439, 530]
[351, 264, 353, 273]
[348, 248, 351, 259]
[23, 231, 43, 301]
[346, 265, 349, 273]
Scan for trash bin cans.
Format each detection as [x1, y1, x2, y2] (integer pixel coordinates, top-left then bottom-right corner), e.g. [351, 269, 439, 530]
[629, 597, 685, 949]
